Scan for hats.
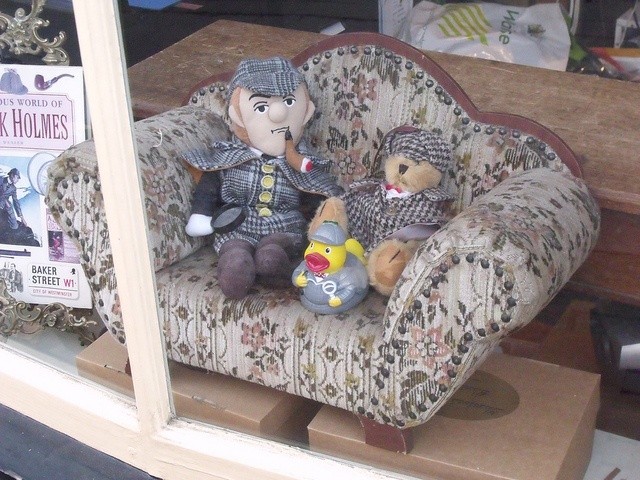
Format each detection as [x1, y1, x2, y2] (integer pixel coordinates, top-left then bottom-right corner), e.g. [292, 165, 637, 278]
[7, 168, 21, 180]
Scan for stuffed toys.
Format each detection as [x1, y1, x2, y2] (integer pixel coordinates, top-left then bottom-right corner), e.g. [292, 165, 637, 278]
[307, 123, 460, 297]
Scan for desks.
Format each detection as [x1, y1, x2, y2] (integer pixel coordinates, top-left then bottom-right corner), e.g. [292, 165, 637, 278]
[125, 16, 639, 307]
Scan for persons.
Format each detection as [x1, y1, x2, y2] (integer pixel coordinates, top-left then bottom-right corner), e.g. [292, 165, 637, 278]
[181, 56, 347, 299]
[0, 168, 28, 230]
[8, 264, 24, 293]
[50, 232, 61, 261]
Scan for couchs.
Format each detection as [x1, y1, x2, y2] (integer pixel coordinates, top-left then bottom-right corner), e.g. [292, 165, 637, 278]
[40, 29, 606, 431]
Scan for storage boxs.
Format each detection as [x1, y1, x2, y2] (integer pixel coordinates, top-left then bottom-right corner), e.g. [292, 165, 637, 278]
[302, 348, 604, 480]
[73, 327, 318, 443]
